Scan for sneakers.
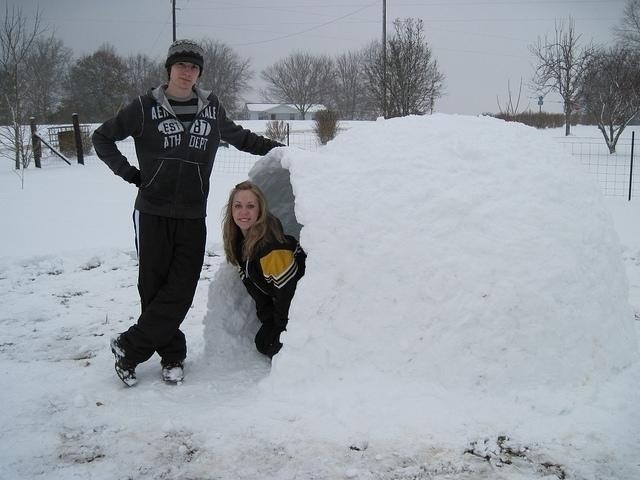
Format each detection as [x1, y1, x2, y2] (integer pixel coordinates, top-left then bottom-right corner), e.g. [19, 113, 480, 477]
[161, 360, 184, 384]
[110, 340, 138, 386]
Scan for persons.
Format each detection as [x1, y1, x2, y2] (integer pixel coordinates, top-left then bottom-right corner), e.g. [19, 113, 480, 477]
[221, 179, 305, 361]
[90, 38, 289, 388]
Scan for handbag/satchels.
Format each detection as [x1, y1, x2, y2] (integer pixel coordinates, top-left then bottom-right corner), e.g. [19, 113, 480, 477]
[256, 295, 279, 321]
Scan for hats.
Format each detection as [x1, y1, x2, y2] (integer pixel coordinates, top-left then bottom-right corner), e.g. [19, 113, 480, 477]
[166, 39, 204, 68]
